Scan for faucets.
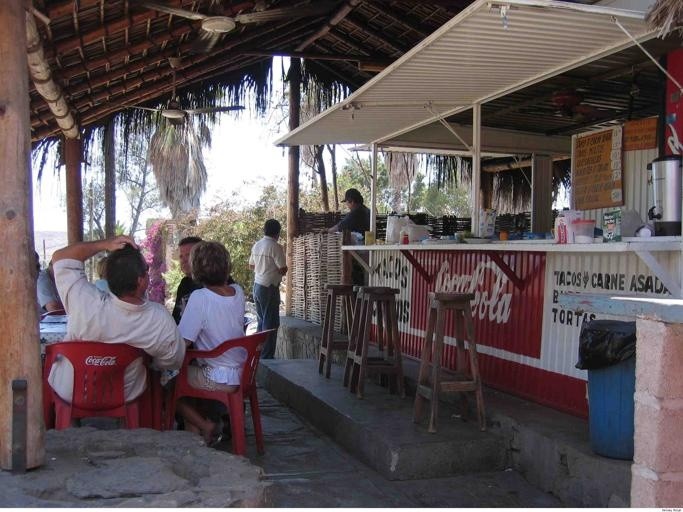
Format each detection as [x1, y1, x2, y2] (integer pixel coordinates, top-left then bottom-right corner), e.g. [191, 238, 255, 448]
[648, 206, 661, 220]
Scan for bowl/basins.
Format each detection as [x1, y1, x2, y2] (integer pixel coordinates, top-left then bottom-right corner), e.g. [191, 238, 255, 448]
[463, 238, 492, 244]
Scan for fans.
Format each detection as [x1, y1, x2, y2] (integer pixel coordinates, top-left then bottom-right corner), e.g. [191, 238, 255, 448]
[97, 0, 303, 124]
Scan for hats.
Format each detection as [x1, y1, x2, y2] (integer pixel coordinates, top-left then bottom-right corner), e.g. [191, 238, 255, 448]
[341, 189, 362, 201]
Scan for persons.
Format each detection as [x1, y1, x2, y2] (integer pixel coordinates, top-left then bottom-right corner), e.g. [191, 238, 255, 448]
[248, 218, 288, 359]
[318, 188, 371, 245]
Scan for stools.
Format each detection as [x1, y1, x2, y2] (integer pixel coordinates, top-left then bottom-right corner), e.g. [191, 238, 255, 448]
[318, 280, 485, 436]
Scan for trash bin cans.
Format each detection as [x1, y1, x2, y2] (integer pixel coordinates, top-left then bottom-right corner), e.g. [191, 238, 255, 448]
[583, 320, 636, 461]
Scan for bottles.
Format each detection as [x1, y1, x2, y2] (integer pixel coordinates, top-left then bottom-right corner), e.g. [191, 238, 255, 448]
[571, 218, 596, 245]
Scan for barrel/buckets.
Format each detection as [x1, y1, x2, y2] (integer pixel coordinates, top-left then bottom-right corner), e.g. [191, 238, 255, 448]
[651, 154, 682, 236]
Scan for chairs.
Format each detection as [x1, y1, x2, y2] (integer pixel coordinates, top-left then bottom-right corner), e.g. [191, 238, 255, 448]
[43, 325, 275, 455]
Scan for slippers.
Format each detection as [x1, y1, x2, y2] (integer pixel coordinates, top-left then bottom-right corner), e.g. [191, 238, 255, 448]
[207, 423, 223, 447]
